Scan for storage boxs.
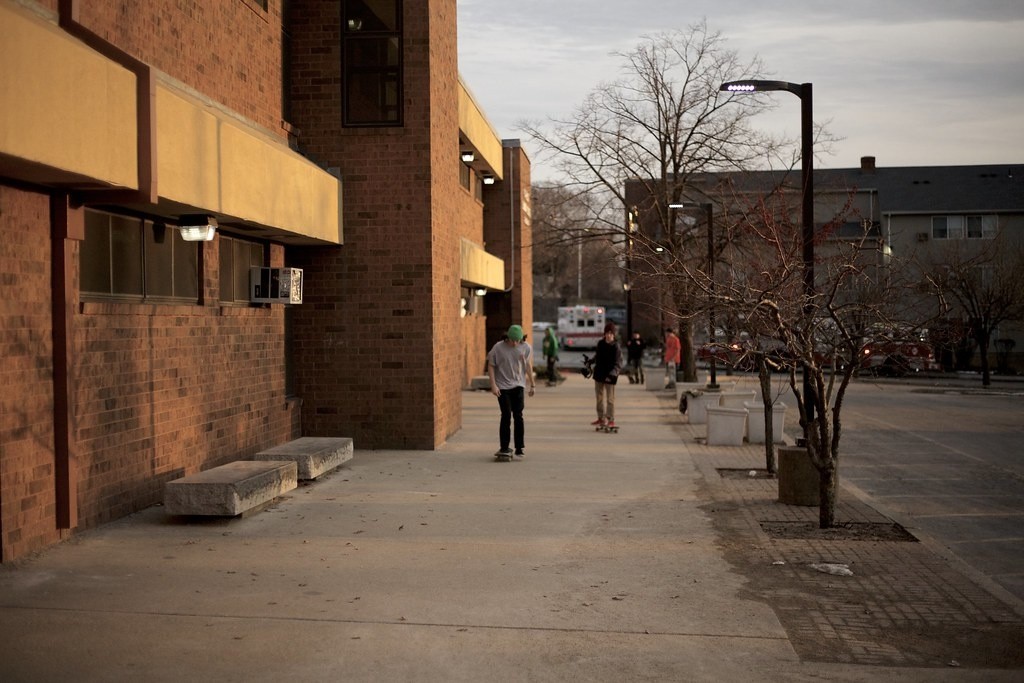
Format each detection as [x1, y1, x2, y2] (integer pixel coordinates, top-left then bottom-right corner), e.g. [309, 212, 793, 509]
[642, 367, 787, 445]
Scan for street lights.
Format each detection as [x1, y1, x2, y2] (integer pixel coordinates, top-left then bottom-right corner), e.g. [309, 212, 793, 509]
[720, 77, 825, 447]
[666, 201, 724, 393]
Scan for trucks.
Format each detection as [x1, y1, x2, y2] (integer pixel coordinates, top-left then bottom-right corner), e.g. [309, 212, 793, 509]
[555, 306, 605, 350]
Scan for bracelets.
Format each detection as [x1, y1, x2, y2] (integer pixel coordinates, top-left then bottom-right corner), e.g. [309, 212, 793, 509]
[531, 385, 535, 389]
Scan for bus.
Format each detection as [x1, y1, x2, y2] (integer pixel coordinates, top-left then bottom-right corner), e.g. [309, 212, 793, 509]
[724, 311, 936, 377]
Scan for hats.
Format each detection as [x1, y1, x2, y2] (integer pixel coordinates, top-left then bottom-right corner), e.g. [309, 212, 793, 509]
[605, 322, 616, 335]
[508, 325, 523, 340]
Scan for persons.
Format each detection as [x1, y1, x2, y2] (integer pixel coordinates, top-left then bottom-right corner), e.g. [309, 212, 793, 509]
[663, 328, 681, 389]
[485, 325, 535, 457]
[542, 327, 558, 388]
[626, 330, 646, 384]
[582, 323, 625, 427]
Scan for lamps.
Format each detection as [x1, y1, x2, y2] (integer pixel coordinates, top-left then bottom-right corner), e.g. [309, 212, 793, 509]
[462, 152, 474, 162]
[483, 176, 495, 184]
[475, 287, 487, 296]
[176, 214, 219, 241]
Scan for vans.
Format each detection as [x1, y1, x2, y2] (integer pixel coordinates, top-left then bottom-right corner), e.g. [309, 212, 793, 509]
[606, 310, 626, 325]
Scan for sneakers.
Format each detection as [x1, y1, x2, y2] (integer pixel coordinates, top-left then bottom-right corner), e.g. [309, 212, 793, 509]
[501, 448, 509, 453]
[515, 448, 525, 457]
[606, 422, 615, 427]
[591, 420, 607, 425]
